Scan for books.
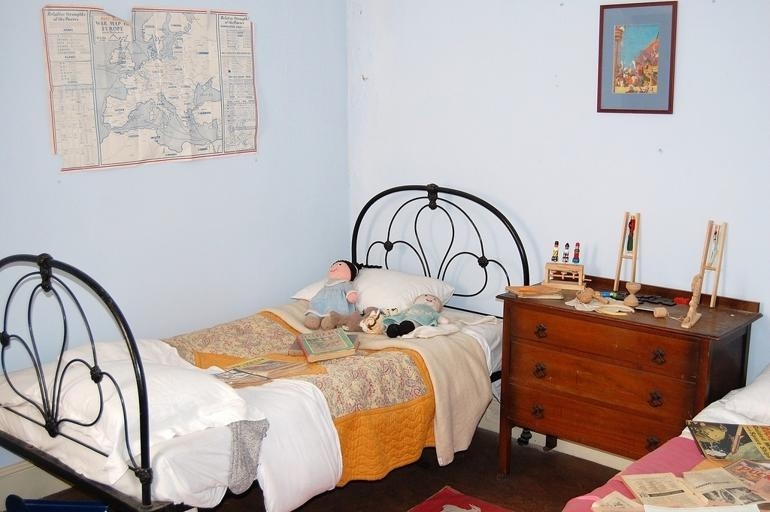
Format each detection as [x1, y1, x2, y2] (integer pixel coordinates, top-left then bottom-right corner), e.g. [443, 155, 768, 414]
[590, 420, 770, 510]
[505, 283, 563, 301]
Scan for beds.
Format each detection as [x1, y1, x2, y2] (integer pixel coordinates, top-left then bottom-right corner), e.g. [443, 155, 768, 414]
[0, 184, 532, 512]
[561, 362, 770, 512]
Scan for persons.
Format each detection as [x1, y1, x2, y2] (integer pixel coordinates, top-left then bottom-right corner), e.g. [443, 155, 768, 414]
[304, 259, 363, 330]
[571, 242, 581, 263]
[382, 293, 450, 338]
[560, 242, 569, 262]
[551, 241, 559, 262]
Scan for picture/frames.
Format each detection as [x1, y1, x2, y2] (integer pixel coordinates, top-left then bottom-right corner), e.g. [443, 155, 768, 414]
[595, 1, 679, 115]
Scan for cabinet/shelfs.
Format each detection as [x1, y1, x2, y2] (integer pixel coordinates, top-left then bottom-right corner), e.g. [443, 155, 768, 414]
[494, 270, 759, 476]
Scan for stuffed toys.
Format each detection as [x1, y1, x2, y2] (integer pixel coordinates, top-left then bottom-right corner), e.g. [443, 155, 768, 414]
[328, 302, 396, 334]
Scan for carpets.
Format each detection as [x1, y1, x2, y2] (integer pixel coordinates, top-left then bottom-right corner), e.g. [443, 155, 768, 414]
[408, 486, 511, 512]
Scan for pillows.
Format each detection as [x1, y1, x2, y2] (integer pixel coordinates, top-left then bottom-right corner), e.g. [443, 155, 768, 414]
[290, 269, 456, 315]
